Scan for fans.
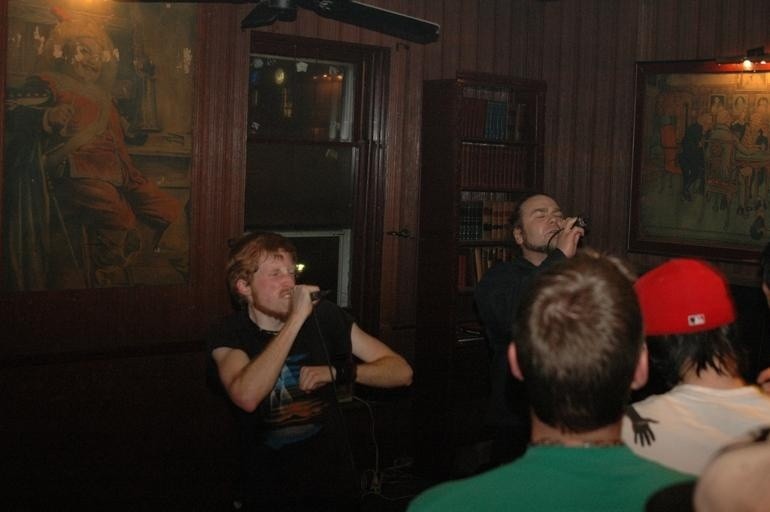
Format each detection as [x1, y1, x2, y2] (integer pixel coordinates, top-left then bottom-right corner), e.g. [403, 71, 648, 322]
[154, 0, 442, 48]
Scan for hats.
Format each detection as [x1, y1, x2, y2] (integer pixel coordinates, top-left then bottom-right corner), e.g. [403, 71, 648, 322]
[631, 257, 737, 337]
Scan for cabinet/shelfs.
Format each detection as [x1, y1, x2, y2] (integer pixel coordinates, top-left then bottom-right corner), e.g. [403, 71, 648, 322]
[413, 73, 545, 462]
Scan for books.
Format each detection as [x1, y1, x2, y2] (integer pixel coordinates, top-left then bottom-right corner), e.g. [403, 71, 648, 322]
[457, 86, 532, 347]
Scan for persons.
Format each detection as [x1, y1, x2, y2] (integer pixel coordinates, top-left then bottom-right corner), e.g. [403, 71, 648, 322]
[471, 192, 587, 471]
[205, 230, 411, 510]
[623, 257, 770, 476]
[693, 437, 768, 512]
[405, 246, 701, 512]
[756, 242, 770, 304]
[1, 17, 191, 268]
[676, 95, 768, 214]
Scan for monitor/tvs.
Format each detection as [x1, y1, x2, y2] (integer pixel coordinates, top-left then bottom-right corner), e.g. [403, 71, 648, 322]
[249, 228, 352, 307]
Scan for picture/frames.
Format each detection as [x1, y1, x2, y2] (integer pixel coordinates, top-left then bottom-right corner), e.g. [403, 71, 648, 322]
[623, 58, 769, 267]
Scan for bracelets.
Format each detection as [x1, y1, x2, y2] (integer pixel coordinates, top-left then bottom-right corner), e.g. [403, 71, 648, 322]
[330, 359, 361, 384]
